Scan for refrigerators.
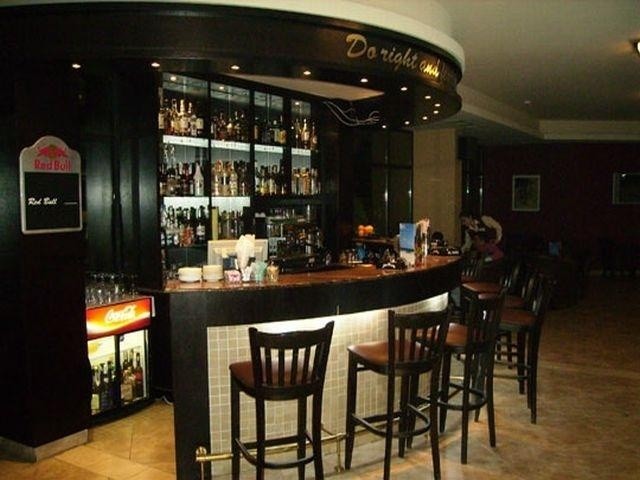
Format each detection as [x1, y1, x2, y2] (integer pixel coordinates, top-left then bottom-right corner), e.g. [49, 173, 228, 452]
[86, 296, 152, 417]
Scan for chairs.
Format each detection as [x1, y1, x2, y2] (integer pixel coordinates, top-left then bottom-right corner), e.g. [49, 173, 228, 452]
[461, 234, 640, 423]
[346, 302, 454, 479]
[407, 296, 505, 465]
[228, 320, 334, 479]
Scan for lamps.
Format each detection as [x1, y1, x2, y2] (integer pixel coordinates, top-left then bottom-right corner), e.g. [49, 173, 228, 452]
[634, 39, 640, 54]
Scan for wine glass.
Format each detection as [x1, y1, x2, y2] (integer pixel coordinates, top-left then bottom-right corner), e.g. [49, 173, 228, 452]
[85, 272, 138, 308]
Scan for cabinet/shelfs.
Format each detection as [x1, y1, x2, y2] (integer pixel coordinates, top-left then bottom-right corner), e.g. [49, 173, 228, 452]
[86, 295, 163, 429]
[119, 65, 329, 292]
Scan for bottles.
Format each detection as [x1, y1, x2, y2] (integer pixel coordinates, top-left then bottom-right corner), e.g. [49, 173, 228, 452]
[91, 348, 144, 413]
[159, 98, 318, 245]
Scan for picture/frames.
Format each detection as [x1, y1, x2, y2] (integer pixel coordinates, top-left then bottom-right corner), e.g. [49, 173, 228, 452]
[612, 172, 640, 205]
[511, 175, 540, 213]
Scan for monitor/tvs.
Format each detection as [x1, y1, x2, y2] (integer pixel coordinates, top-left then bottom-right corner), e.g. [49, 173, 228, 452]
[207, 239, 268, 272]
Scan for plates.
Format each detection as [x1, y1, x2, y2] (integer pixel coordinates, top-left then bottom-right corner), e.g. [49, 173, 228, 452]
[179, 264, 224, 283]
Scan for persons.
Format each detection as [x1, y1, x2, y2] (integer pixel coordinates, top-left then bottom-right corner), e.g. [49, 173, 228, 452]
[447, 232, 503, 320]
[459, 212, 502, 254]
[428, 230, 449, 255]
